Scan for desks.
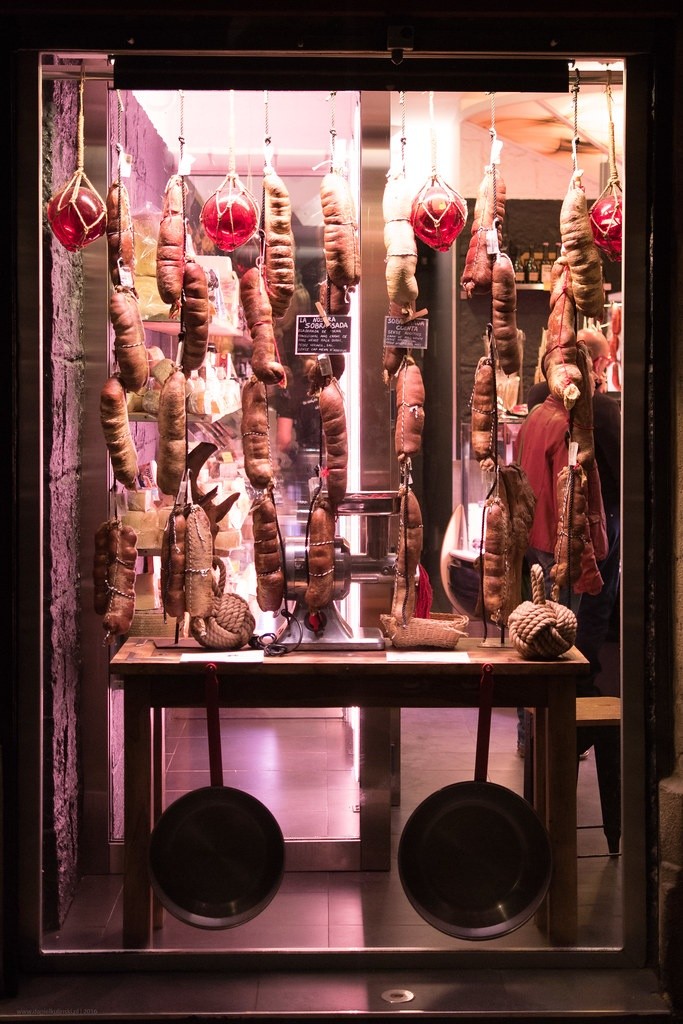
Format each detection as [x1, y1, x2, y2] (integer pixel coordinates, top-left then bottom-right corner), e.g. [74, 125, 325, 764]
[106, 634, 594, 950]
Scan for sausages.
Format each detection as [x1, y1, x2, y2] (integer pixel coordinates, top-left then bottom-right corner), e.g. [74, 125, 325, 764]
[98, 172, 620, 633]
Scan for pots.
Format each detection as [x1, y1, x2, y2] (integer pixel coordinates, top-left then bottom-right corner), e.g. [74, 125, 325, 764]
[145, 662, 287, 931]
[397, 661, 554, 940]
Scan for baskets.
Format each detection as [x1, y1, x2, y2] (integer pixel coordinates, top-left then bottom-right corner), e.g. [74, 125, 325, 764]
[380, 610, 469, 647]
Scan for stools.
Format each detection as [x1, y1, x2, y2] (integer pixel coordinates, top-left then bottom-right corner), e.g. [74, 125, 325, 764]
[520, 694, 623, 866]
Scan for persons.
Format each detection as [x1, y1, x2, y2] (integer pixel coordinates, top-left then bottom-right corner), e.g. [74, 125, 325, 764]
[510, 346, 609, 759]
[528, 328, 620, 530]
